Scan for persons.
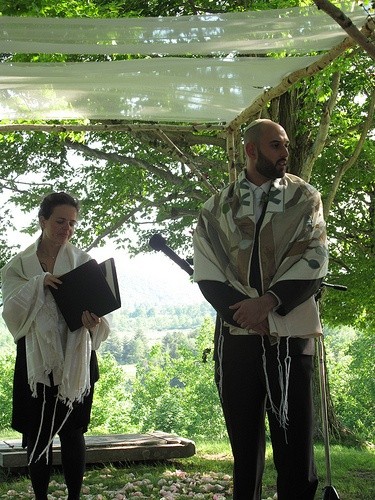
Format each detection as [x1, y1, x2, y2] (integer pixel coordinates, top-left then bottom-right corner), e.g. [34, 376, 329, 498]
[0, 192, 111, 500]
[193, 119, 329, 500]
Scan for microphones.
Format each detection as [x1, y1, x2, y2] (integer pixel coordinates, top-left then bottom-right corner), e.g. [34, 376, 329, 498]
[150, 235, 194, 276]
[320, 282, 348, 291]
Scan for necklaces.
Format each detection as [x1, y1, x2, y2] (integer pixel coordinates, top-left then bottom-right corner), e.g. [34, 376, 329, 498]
[39, 246, 60, 263]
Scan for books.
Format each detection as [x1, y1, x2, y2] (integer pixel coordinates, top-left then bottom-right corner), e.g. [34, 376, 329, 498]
[48, 257, 121, 332]
